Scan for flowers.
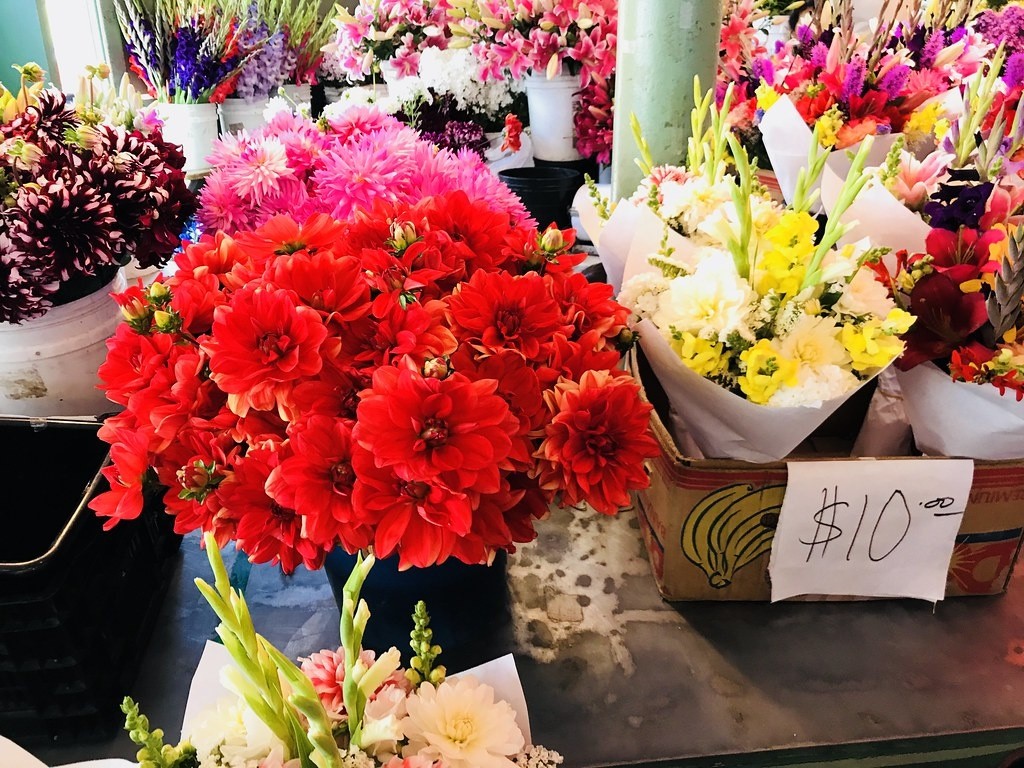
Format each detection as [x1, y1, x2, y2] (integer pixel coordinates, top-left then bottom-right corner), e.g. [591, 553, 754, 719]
[1, 1, 1024, 768]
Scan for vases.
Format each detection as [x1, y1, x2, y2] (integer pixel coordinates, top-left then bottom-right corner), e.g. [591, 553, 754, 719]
[0, 272, 127, 423]
[525, 75, 589, 161]
[268, 82, 312, 116]
[155, 103, 216, 172]
[326, 540, 511, 643]
[758, 168, 784, 205]
[494, 168, 577, 236]
[323, 85, 388, 111]
[485, 131, 537, 171]
[216, 97, 269, 138]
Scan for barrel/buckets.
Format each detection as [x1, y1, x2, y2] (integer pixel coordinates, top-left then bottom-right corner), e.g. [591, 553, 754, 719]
[157, 101, 220, 172]
[522, 74, 596, 162]
[480, 130, 536, 185]
[378, 57, 413, 103]
[124, 252, 164, 293]
[323, 83, 361, 108]
[756, 163, 793, 207]
[266, 81, 313, 130]
[0, 265, 135, 420]
[497, 166, 582, 235]
[532, 148, 601, 189]
[322, 540, 513, 656]
[217, 95, 268, 146]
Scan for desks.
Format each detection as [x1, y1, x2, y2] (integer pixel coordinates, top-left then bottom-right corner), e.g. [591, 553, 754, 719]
[0, 416, 112, 574]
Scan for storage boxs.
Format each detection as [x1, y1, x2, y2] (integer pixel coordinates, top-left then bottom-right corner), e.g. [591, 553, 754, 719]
[624, 340, 1024, 606]
